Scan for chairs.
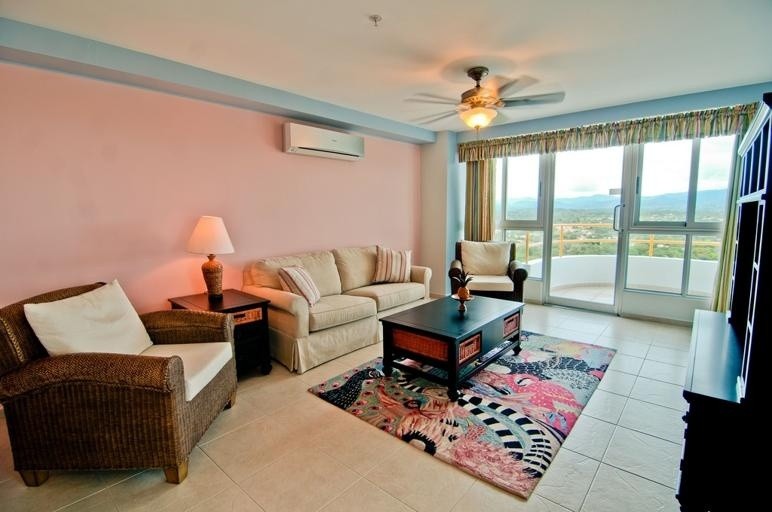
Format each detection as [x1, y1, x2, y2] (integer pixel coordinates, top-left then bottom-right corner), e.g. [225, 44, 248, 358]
[1, 279, 239, 483]
[449, 238, 529, 304]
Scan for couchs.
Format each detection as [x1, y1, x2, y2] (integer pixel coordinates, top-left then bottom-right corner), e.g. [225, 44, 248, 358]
[241, 245, 432, 376]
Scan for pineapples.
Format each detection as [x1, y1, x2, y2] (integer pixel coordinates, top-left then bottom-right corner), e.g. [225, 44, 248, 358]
[458, 287, 470, 299]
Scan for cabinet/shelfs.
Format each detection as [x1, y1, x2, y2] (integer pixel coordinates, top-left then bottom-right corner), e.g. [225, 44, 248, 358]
[672, 92, 772, 510]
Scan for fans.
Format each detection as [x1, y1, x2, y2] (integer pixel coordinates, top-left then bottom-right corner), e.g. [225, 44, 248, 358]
[404, 64, 567, 129]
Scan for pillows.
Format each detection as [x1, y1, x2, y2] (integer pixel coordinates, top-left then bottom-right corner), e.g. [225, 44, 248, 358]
[370, 245, 414, 285]
[277, 265, 322, 307]
[19, 279, 153, 359]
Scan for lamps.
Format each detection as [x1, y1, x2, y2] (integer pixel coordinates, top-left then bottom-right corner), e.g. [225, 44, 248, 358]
[457, 102, 497, 133]
[186, 214, 235, 296]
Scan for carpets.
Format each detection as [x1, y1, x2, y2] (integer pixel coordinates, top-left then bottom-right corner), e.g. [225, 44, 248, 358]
[307, 329, 618, 503]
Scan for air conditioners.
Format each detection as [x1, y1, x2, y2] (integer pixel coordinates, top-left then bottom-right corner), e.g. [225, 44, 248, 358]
[282, 121, 367, 163]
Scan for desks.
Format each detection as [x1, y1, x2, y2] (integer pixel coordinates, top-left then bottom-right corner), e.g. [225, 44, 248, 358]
[166, 288, 273, 381]
